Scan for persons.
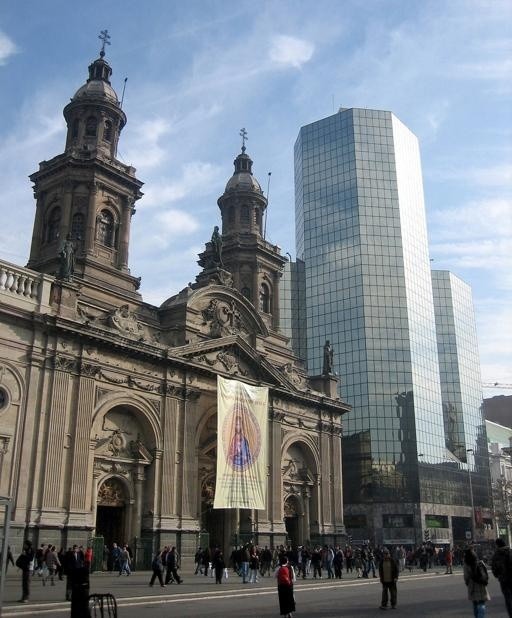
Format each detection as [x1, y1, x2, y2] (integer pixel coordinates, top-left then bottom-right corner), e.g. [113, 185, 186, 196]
[103, 542, 133, 577]
[211, 225, 225, 268]
[149, 546, 183, 587]
[227, 412, 253, 469]
[194, 547, 226, 584]
[462, 549, 491, 617]
[6, 539, 92, 602]
[323, 339, 334, 376]
[231, 540, 479, 584]
[54, 231, 81, 283]
[273, 554, 297, 618]
[377, 547, 400, 611]
[489, 536, 512, 617]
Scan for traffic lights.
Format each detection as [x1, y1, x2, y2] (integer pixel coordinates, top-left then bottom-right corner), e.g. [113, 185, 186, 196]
[347, 535, 353, 543]
[424, 528, 430, 540]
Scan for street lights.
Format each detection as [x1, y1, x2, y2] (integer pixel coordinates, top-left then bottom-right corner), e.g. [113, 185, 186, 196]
[465, 446, 477, 544]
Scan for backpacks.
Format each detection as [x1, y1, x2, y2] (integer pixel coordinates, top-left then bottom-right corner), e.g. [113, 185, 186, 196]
[278, 563, 291, 585]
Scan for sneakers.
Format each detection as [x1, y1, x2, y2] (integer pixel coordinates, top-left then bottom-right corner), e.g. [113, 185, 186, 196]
[380, 606, 386, 610]
[393, 605, 396, 608]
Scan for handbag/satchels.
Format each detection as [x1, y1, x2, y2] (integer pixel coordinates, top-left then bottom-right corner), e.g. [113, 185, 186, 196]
[474, 564, 488, 585]
